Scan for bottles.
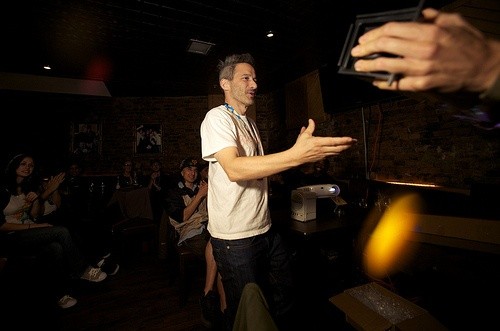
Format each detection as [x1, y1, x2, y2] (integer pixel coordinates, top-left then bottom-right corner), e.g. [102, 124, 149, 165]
[99, 177, 106, 194]
[116, 176, 121, 189]
[132, 172, 136, 185]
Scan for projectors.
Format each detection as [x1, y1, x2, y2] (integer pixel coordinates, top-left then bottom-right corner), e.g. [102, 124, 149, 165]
[296, 184, 341, 199]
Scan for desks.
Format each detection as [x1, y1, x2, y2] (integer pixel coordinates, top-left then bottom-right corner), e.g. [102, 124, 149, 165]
[107, 182, 164, 220]
[286, 218, 351, 331]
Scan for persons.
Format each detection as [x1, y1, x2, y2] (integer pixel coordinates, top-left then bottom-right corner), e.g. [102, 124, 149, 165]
[351, 8, 500, 99]
[119, 161, 137, 188]
[200, 55, 352, 331]
[148, 159, 164, 224]
[165, 158, 232, 328]
[0, 154, 120, 309]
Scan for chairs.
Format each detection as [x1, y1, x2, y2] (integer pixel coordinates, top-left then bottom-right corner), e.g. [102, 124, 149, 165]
[163, 196, 205, 301]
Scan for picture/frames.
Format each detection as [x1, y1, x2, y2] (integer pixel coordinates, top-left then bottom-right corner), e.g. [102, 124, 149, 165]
[66, 117, 104, 164]
[131, 119, 169, 162]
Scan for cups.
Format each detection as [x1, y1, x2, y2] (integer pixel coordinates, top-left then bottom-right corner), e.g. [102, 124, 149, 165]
[88, 175, 95, 193]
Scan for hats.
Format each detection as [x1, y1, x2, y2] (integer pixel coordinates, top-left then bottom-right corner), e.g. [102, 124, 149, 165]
[180, 157, 201, 172]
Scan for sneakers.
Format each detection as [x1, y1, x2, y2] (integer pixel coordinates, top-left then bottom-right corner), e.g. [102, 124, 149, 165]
[59, 295, 77, 308]
[80, 253, 119, 282]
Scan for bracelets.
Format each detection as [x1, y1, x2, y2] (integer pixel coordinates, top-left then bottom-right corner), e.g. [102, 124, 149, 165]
[28, 224, 30, 230]
